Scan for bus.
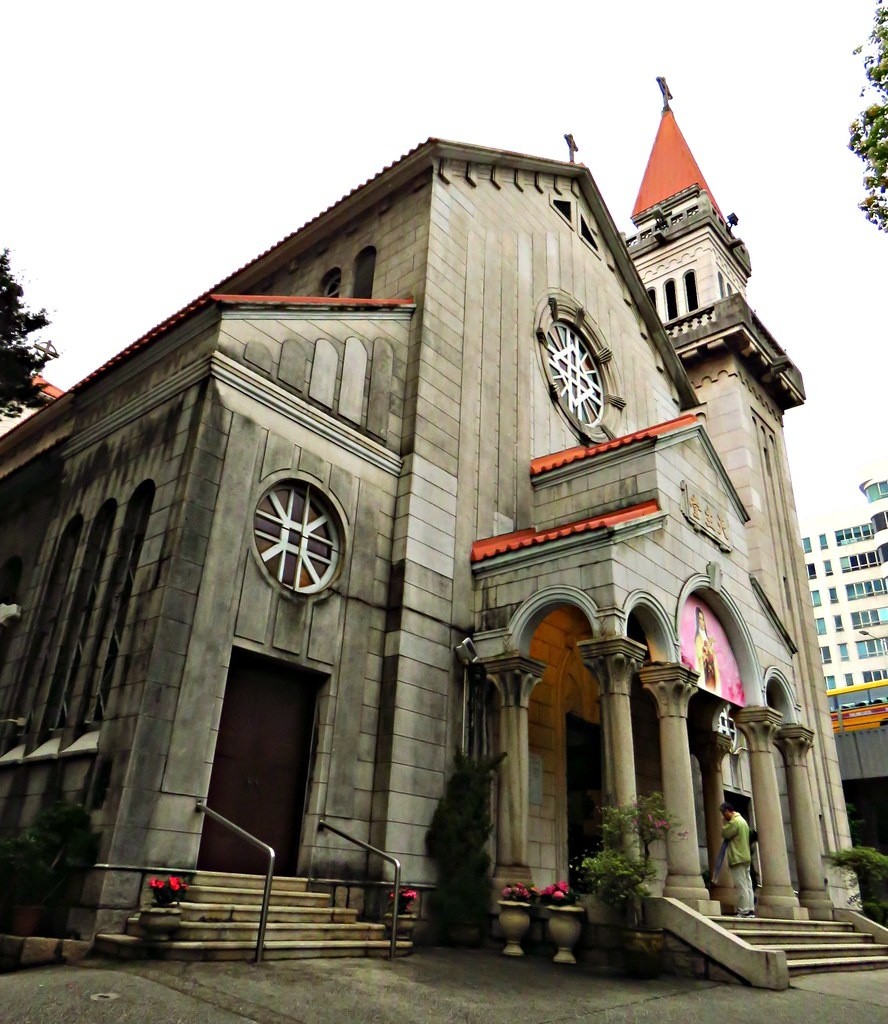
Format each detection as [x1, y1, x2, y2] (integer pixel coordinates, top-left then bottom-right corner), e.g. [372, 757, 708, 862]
[826, 680, 888, 733]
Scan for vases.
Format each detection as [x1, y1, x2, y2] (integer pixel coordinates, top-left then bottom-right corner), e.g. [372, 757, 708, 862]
[384, 913, 414, 941]
[641, 878, 663, 896]
[498, 897, 533, 957]
[137, 905, 181, 942]
[542, 903, 585, 965]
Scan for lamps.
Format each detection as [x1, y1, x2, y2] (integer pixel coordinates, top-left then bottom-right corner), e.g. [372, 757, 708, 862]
[0, 601, 21, 626]
[727, 212, 740, 232]
[455, 638, 480, 665]
[650, 207, 672, 229]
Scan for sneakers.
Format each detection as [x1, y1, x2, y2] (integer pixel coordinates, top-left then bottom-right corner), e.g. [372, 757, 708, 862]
[737, 913, 755, 918]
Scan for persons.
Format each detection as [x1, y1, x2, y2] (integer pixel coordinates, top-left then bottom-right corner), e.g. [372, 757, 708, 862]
[719, 802, 755, 920]
[693, 606, 722, 699]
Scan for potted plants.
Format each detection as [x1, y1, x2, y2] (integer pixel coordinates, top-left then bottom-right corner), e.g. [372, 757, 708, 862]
[570, 848, 669, 980]
[429, 749, 509, 947]
[0, 800, 103, 936]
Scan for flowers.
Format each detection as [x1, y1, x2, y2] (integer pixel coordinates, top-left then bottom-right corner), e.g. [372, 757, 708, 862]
[537, 879, 587, 907]
[597, 790, 689, 878]
[385, 887, 417, 916]
[499, 879, 537, 902]
[144, 874, 189, 908]
